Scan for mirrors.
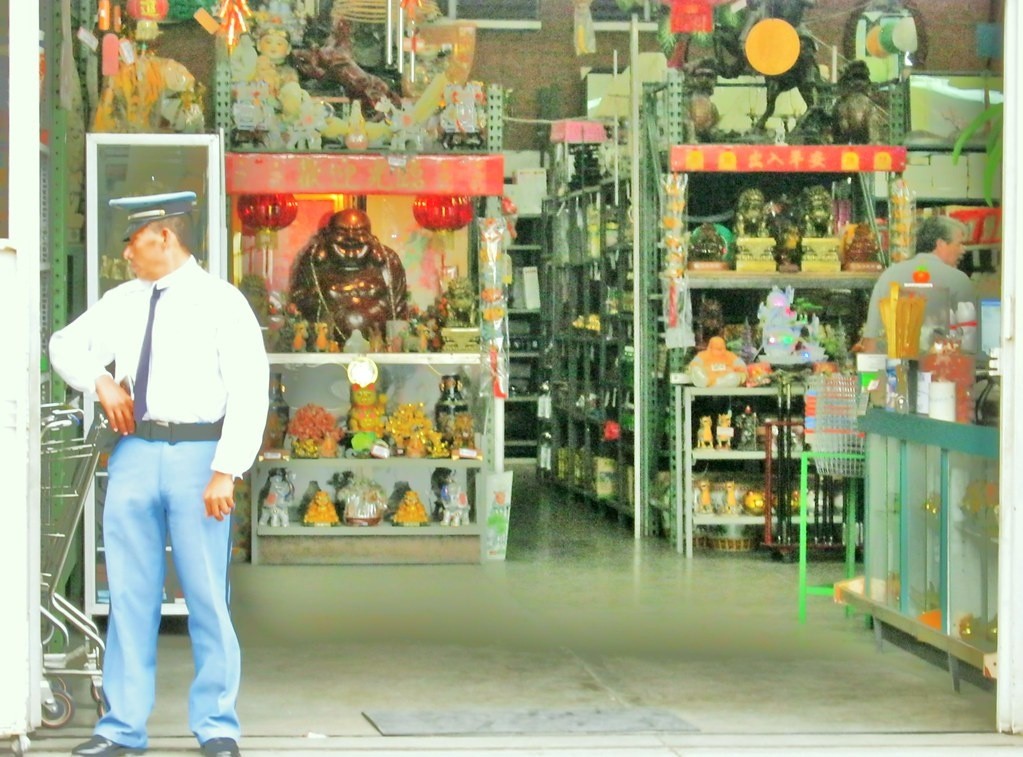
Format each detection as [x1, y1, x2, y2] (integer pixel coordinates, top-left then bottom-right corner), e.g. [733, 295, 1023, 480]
[83, 127, 226, 614]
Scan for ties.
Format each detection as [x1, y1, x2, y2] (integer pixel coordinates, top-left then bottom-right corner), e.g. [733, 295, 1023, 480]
[134, 284, 167, 424]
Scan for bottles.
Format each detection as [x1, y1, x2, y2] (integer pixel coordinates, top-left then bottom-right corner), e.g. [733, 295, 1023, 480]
[881, 300, 977, 425]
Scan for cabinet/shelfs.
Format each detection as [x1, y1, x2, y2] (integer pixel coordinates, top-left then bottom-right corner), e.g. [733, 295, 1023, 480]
[250, 321, 505, 566]
[538, 73, 911, 627]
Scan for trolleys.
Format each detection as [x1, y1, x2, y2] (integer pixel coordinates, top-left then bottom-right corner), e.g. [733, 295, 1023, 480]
[38, 401, 109, 731]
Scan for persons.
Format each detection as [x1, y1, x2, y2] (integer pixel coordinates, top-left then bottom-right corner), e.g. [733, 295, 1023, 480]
[737, 404, 759, 449]
[49, 191, 270, 757]
[289, 210, 410, 342]
[860, 215, 978, 360]
[255, 23, 302, 100]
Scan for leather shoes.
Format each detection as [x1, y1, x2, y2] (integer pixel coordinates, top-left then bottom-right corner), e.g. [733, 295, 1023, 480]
[200, 737, 241, 757]
[71, 734, 149, 757]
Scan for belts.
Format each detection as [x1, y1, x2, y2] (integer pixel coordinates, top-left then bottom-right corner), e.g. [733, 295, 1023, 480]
[134, 415, 225, 445]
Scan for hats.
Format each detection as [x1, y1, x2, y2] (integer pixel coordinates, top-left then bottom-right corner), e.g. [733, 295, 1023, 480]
[108, 191, 198, 241]
